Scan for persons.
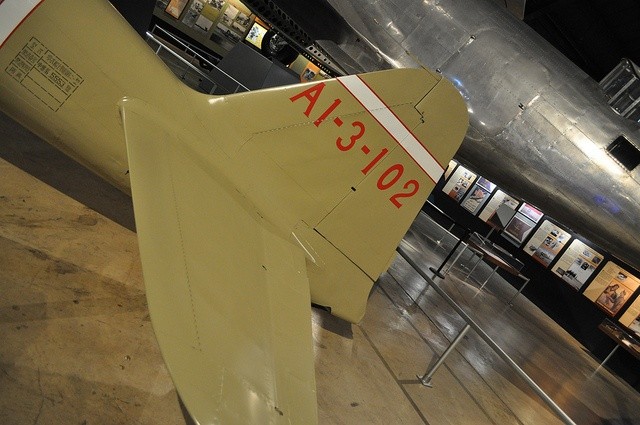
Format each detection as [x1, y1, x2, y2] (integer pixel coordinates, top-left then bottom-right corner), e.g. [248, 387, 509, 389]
[598, 284, 619, 308]
[608, 290, 626, 313]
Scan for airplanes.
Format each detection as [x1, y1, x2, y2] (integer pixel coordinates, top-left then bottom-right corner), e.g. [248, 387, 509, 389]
[0, 0, 471, 425]
